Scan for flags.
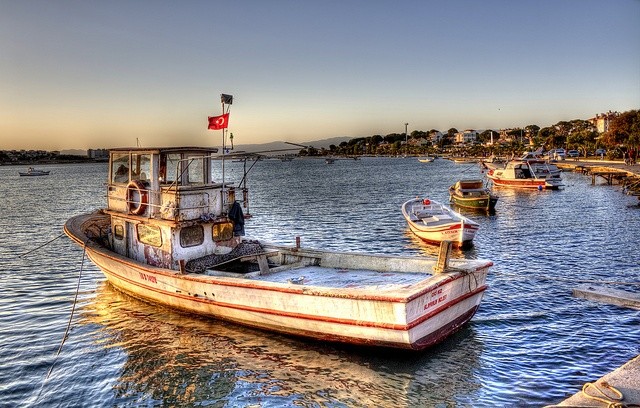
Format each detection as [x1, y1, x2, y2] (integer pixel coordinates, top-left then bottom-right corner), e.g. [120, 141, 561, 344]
[208, 113, 229, 130]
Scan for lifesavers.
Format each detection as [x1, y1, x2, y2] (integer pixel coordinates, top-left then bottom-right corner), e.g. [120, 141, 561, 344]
[125, 180, 147, 216]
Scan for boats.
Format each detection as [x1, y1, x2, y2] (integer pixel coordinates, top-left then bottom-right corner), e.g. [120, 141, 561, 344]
[19, 167, 51, 176]
[60, 93, 493, 351]
[486, 156, 565, 191]
[448, 178, 499, 216]
[418, 158, 434, 163]
[401, 197, 480, 250]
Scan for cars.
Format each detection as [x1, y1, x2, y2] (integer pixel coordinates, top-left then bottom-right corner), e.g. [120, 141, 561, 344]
[522, 147, 566, 157]
[587, 149, 607, 157]
[567, 150, 579, 157]
